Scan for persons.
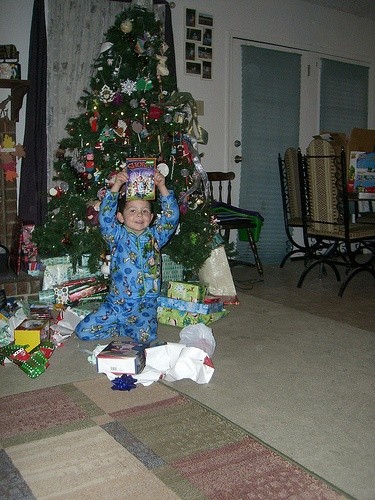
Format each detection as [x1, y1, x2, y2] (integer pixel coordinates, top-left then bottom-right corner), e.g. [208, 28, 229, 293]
[75, 167, 180, 344]
[188, 14, 212, 77]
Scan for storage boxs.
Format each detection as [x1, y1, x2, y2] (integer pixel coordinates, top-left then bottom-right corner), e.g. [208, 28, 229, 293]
[157, 294, 224, 313]
[95, 339, 150, 376]
[13, 317, 52, 350]
[167, 278, 208, 300]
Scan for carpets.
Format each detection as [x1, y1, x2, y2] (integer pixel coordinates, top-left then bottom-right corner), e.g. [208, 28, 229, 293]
[0, 288, 375, 500]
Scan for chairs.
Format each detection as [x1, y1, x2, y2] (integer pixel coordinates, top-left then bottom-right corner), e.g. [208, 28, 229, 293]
[276, 145, 354, 277]
[203, 168, 268, 282]
[294, 137, 375, 301]
